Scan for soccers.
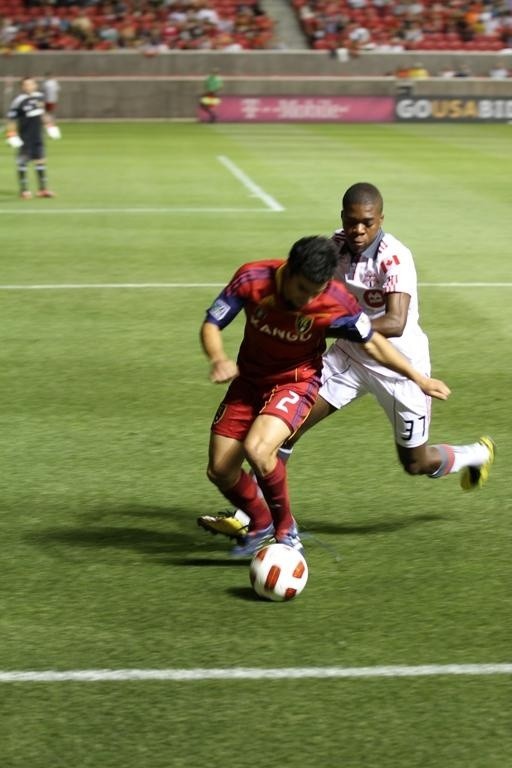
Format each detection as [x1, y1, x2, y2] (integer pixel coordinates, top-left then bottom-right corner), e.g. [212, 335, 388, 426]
[250, 544, 308, 601]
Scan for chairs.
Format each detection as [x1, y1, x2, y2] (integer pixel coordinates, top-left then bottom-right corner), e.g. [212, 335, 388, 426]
[0, 0, 512, 50]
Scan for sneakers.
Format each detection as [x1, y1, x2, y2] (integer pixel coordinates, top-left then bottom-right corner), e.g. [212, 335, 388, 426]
[461, 435, 496, 491]
[197, 509, 305, 557]
[20, 189, 53, 201]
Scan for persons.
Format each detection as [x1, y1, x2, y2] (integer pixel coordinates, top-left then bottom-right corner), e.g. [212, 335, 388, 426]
[44, 72, 60, 111]
[2, 75, 64, 201]
[195, 180, 499, 548]
[206, 72, 223, 96]
[198, 233, 452, 557]
[395, 63, 510, 77]
[1, 0, 512, 52]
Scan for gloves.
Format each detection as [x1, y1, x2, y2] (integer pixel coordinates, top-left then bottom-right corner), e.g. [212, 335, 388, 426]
[5, 131, 24, 148]
[47, 123, 61, 139]
